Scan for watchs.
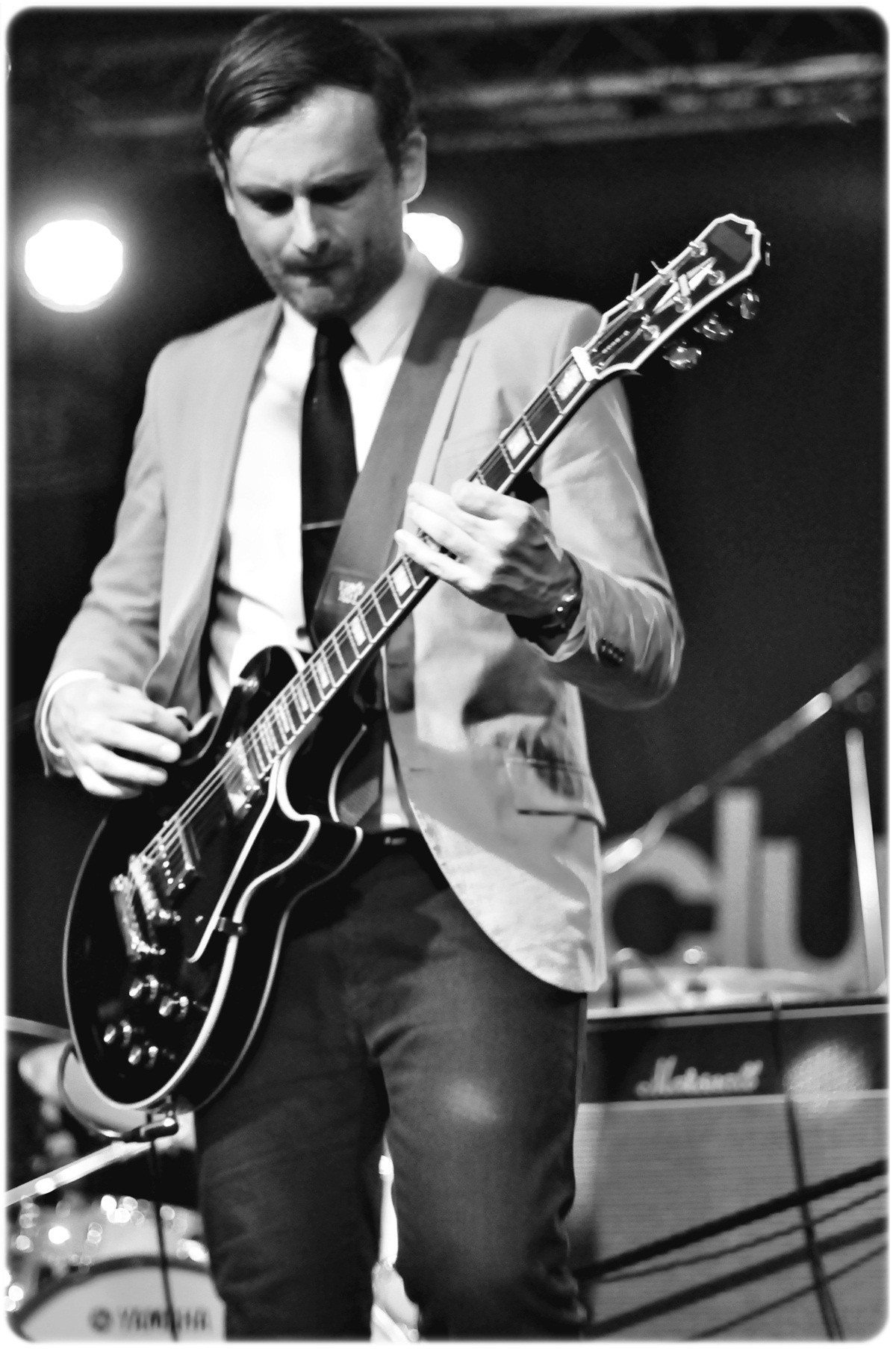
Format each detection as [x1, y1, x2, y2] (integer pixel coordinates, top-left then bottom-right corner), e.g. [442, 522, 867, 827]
[522, 557, 580, 639]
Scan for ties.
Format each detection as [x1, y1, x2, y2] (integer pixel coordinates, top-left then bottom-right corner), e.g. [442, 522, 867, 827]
[302, 324, 387, 821]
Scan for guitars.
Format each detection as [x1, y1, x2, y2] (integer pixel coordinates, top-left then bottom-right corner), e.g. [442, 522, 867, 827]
[58, 204, 779, 1114]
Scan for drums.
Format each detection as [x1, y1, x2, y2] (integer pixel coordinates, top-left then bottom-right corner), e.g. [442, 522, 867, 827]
[6, 1191, 412, 1341]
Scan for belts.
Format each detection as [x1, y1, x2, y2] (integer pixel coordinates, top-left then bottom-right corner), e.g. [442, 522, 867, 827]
[338, 827, 428, 873]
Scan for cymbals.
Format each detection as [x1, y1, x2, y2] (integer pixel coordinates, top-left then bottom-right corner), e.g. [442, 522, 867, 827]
[17, 1036, 197, 1137]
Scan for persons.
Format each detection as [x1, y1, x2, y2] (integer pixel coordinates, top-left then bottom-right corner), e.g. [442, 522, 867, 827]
[32, 9, 685, 1339]
